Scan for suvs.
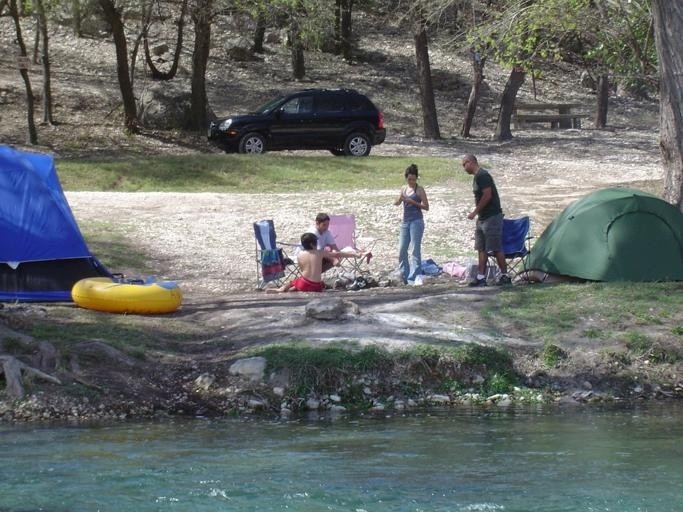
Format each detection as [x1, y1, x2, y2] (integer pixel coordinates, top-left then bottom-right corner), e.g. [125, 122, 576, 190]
[206, 86, 387, 158]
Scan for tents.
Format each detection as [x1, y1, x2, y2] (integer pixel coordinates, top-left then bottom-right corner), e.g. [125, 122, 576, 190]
[510, 186, 682, 283]
[0, 140, 117, 303]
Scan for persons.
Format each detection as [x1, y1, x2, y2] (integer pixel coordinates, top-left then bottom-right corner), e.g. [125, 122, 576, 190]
[263, 232, 339, 294]
[392, 162, 429, 286]
[294, 211, 344, 276]
[461, 153, 511, 288]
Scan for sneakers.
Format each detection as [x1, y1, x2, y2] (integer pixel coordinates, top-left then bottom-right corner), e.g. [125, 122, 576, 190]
[496, 275, 512, 284]
[468, 276, 487, 286]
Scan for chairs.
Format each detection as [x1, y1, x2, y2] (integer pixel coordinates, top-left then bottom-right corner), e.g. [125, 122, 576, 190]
[484, 214, 533, 286]
[252, 212, 369, 289]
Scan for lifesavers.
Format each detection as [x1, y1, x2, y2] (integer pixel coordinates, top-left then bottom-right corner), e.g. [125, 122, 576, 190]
[71, 276, 182, 313]
